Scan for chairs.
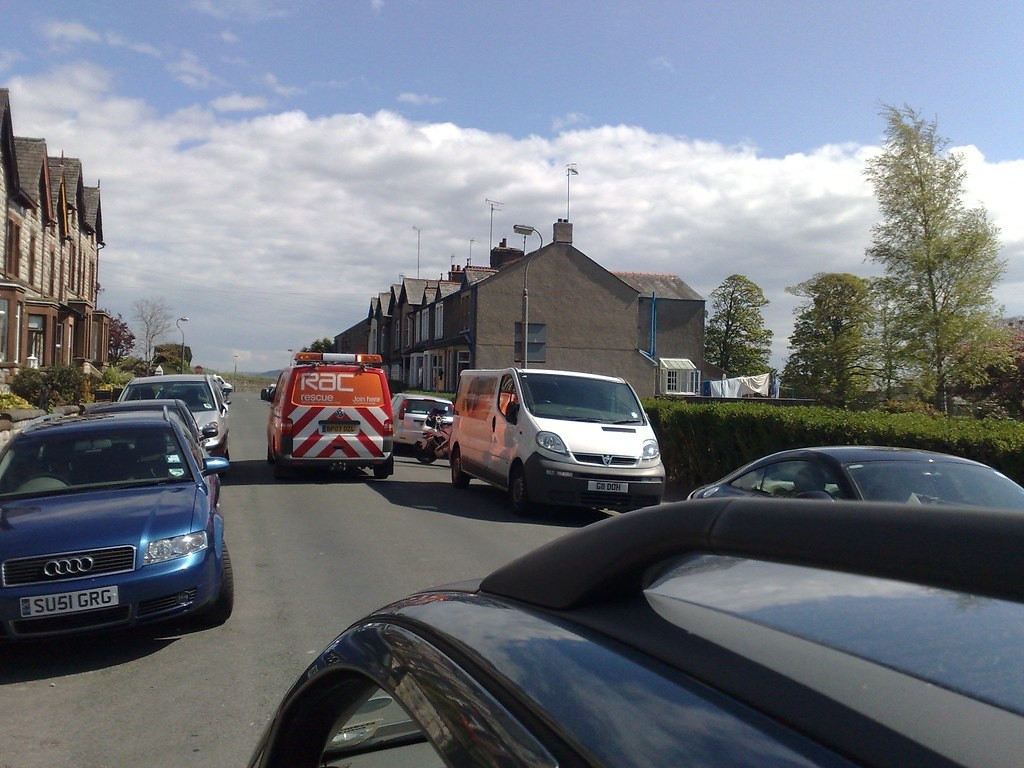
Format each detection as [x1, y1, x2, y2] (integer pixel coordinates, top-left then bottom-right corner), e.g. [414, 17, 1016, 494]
[793, 466, 833, 499]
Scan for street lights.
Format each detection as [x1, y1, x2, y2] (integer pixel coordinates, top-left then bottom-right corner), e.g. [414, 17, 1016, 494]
[233, 354, 239, 392]
[512, 223, 544, 369]
[176, 316, 189, 374]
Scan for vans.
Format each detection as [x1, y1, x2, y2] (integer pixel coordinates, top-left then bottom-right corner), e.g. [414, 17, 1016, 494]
[447, 365, 666, 520]
[265, 351, 396, 479]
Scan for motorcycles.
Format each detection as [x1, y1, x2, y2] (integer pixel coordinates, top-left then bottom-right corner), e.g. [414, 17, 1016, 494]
[411, 406, 453, 464]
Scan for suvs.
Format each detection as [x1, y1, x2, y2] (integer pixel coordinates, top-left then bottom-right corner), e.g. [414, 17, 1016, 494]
[244, 493, 1024, 768]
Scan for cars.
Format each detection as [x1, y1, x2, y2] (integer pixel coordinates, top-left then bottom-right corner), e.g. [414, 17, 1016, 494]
[0, 404, 236, 658]
[260, 384, 276, 402]
[73, 398, 221, 507]
[206, 373, 232, 398]
[390, 393, 455, 454]
[116, 372, 232, 476]
[683, 446, 1024, 512]
